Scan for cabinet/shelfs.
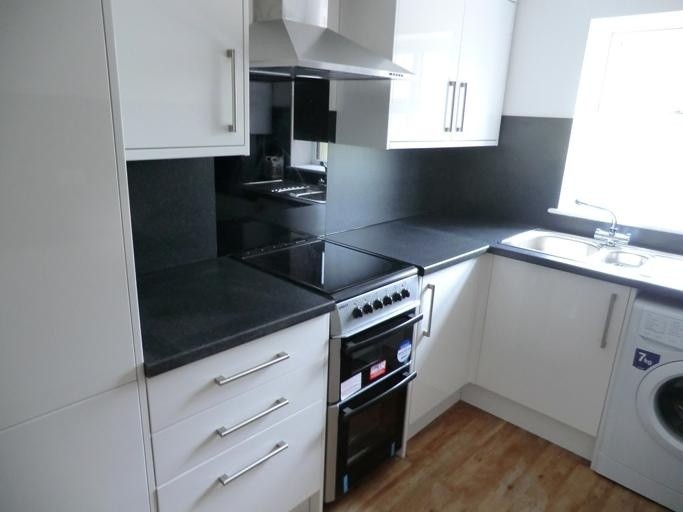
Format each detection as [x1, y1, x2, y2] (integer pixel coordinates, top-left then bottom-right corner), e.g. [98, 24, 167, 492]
[109, 0, 251, 163]
[336, 0, 516, 151]
[0, 4, 152, 512]
[476, 255, 630, 441]
[407, 253, 485, 420]
[142, 312, 330, 511]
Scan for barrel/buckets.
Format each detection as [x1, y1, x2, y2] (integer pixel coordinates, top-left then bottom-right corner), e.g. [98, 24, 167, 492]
[263, 156, 269, 180]
[269, 156, 285, 180]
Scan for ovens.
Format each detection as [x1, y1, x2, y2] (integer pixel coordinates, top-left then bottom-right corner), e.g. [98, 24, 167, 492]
[323, 299, 424, 504]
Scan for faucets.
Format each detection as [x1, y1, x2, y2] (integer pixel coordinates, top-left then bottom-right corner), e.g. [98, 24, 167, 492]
[576, 198, 616, 234]
[296, 191, 326, 203]
[319, 162, 327, 183]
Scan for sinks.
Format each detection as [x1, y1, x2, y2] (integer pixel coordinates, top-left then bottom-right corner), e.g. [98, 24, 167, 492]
[499, 226, 599, 266]
[602, 250, 650, 268]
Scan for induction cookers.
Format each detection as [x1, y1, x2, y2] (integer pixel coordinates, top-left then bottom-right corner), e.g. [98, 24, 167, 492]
[242, 236, 412, 302]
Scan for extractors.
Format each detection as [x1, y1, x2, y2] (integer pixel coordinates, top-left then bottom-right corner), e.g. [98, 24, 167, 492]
[248, 0, 414, 84]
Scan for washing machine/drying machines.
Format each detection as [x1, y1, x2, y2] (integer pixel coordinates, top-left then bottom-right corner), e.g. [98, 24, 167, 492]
[593, 291, 683, 511]
